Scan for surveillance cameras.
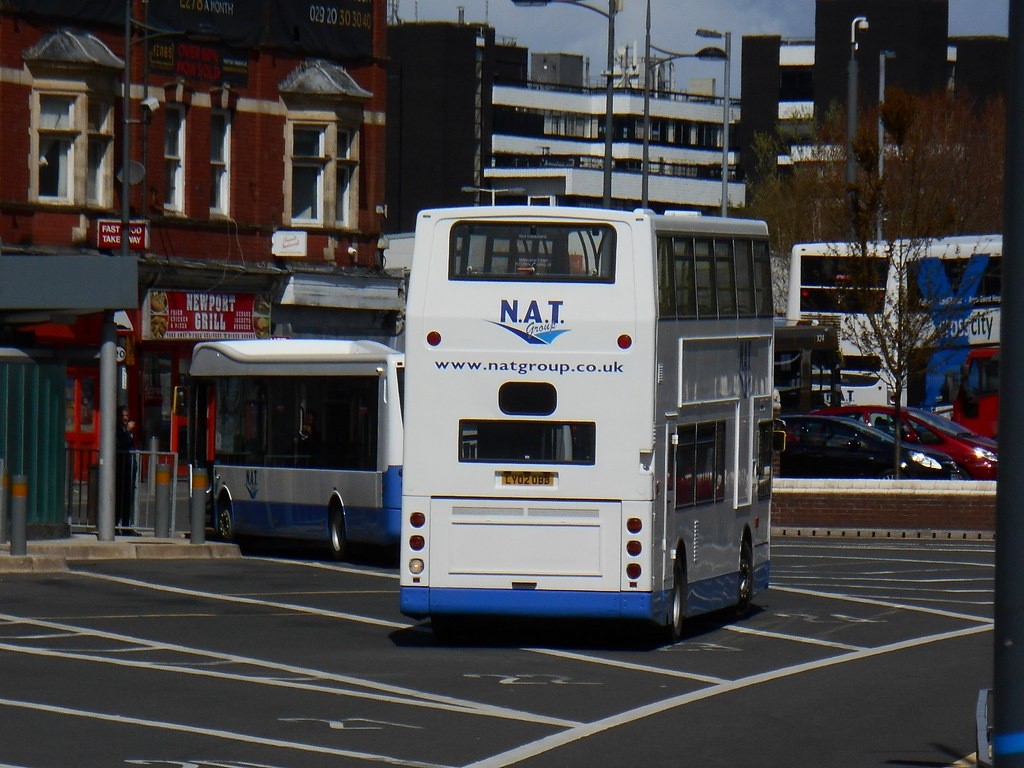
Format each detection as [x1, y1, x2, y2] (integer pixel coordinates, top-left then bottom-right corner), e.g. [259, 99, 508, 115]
[859, 21, 869, 33]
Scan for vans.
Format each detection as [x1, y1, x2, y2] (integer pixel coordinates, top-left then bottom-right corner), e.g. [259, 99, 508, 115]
[951, 347, 1003, 443]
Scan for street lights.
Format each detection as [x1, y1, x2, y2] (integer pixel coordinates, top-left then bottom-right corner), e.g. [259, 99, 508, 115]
[877, 49, 897, 242]
[695, 29, 731, 218]
[511, 0, 617, 208]
[642, 0, 728, 210]
[847, 17, 869, 242]
[121, 0, 223, 256]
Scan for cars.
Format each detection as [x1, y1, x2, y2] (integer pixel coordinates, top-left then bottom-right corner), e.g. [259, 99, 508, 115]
[773, 416, 971, 480]
[786, 406, 998, 480]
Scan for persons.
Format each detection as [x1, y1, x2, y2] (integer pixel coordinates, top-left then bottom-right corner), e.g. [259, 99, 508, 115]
[116, 405, 141, 537]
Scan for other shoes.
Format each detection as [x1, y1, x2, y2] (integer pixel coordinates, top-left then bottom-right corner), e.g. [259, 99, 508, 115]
[122, 529, 141, 536]
[114, 528, 121, 537]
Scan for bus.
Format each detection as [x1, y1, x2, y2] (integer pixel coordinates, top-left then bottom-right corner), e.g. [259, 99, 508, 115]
[785, 234, 1003, 406]
[400, 205, 776, 645]
[190, 338, 405, 561]
